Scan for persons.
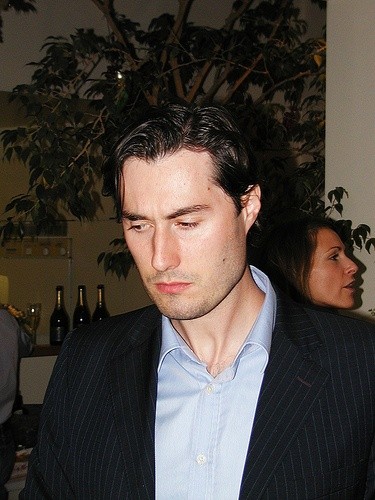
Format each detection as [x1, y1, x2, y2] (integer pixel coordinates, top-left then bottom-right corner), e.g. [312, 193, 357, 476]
[17, 103, 375, 500]
[254, 206, 360, 307]
[0, 307, 25, 500]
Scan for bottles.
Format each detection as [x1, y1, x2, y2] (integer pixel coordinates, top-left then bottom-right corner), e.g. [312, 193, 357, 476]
[50, 285, 70, 344]
[73, 285, 90, 329]
[92, 285, 109, 322]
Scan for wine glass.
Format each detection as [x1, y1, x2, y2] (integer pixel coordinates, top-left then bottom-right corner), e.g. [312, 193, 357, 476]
[26, 302, 42, 344]
